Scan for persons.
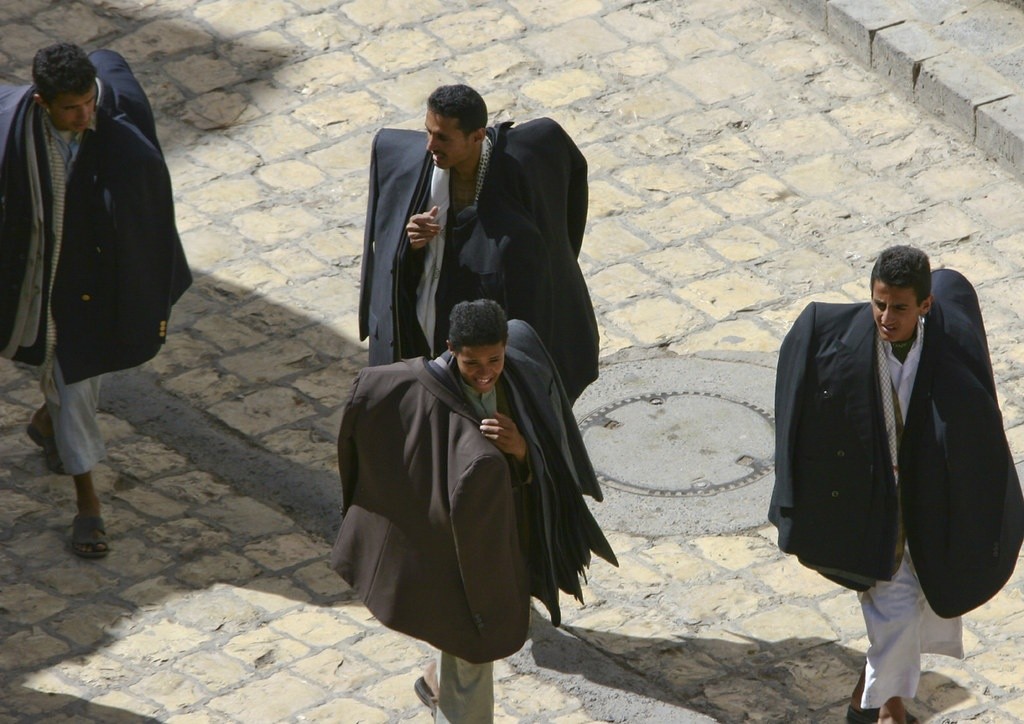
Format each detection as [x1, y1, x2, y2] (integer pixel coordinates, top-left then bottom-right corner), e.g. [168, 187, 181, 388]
[1, 40, 198, 561]
[355, 80, 605, 414]
[767, 242, 1023, 724]
[326, 296, 624, 724]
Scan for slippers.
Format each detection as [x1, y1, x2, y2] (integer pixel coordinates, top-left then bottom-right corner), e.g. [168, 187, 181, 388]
[846, 701, 919, 724]
[70, 512, 110, 559]
[24, 423, 68, 475]
[414, 676, 441, 724]
